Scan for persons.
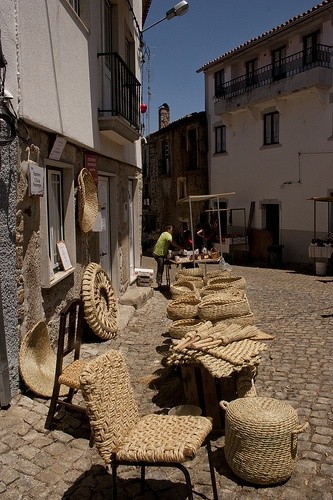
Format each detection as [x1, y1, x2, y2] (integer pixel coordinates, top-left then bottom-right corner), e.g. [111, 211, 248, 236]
[197, 217, 219, 251]
[152, 226, 180, 291]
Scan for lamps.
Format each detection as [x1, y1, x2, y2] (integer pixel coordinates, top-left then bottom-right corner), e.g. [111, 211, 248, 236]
[139, 0, 189, 47]
[143, 195, 151, 208]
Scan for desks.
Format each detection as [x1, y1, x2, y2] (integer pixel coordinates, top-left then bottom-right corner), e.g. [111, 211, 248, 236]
[308, 245, 333, 265]
[213, 243, 249, 260]
[166, 257, 221, 288]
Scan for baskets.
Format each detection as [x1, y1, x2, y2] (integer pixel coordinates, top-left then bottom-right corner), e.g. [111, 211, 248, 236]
[167, 268, 254, 339]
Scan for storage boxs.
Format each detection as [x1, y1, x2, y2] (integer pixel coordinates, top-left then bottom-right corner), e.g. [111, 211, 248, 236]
[225, 237, 242, 245]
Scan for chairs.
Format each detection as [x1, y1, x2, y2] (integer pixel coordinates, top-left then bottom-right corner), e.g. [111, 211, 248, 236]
[44, 299, 102, 448]
[81, 349, 218, 500]
